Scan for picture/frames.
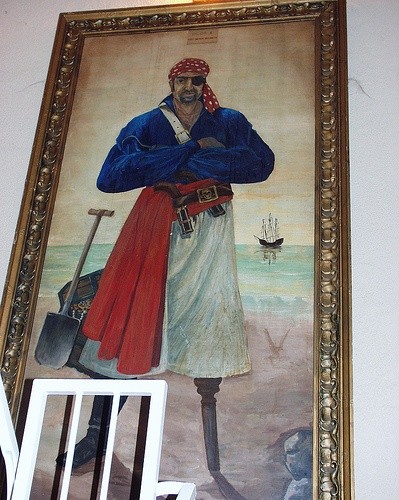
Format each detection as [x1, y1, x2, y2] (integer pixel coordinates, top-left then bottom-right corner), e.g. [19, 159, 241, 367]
[0, 0, 355, 500]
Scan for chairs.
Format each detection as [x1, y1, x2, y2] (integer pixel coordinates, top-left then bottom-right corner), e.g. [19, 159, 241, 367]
[0, 374, 197, 500]
